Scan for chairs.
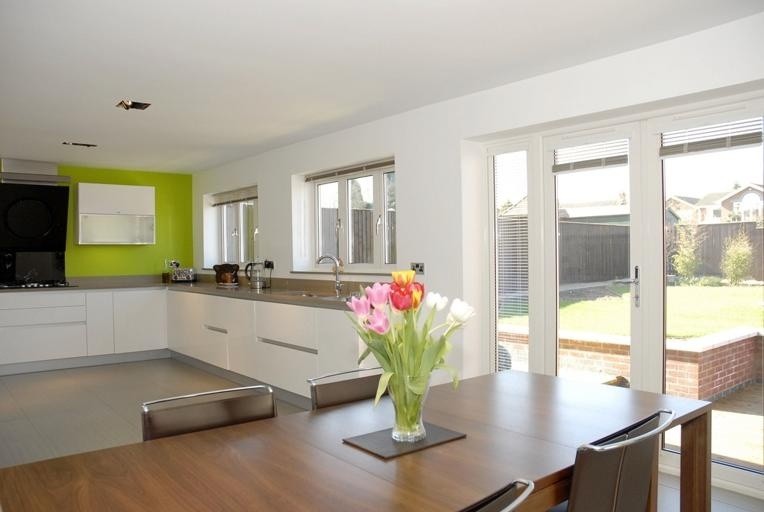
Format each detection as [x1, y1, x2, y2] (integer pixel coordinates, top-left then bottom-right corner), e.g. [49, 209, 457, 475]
[457, 479, 535, 512]
[306, 369, 393, 410]
[140, 384, 278, 441]
[567, 407, 679, 511]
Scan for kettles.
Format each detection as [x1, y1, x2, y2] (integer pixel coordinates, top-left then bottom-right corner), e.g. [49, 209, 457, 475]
[245, 263, 266, 289]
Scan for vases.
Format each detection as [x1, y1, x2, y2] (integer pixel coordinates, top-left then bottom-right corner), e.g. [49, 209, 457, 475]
[386, 375, 433, 444]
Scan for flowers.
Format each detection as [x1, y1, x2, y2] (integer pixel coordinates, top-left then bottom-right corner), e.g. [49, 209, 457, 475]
[344, 269, 476, 434]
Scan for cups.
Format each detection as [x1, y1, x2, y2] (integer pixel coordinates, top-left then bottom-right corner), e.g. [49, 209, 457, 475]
[225, 273, 232, 283]
[162, 272, 170, 283]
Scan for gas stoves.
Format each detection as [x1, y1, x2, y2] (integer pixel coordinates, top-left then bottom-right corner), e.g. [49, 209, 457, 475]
[0, 277, 79, 289]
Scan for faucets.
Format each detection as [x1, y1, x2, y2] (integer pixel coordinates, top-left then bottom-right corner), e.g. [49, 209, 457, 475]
[316, 254, 344, 297]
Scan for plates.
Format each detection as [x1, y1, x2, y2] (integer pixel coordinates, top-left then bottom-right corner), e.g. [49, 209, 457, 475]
[219, 283, 238, 286]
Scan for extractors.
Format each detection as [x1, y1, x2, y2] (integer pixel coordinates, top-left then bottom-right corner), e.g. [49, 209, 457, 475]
[0, 157, 71, 253]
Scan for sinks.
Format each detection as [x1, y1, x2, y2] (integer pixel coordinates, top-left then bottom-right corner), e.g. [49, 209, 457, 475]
[288, 292, 320, 299]
[323, 297, 349, 301]
